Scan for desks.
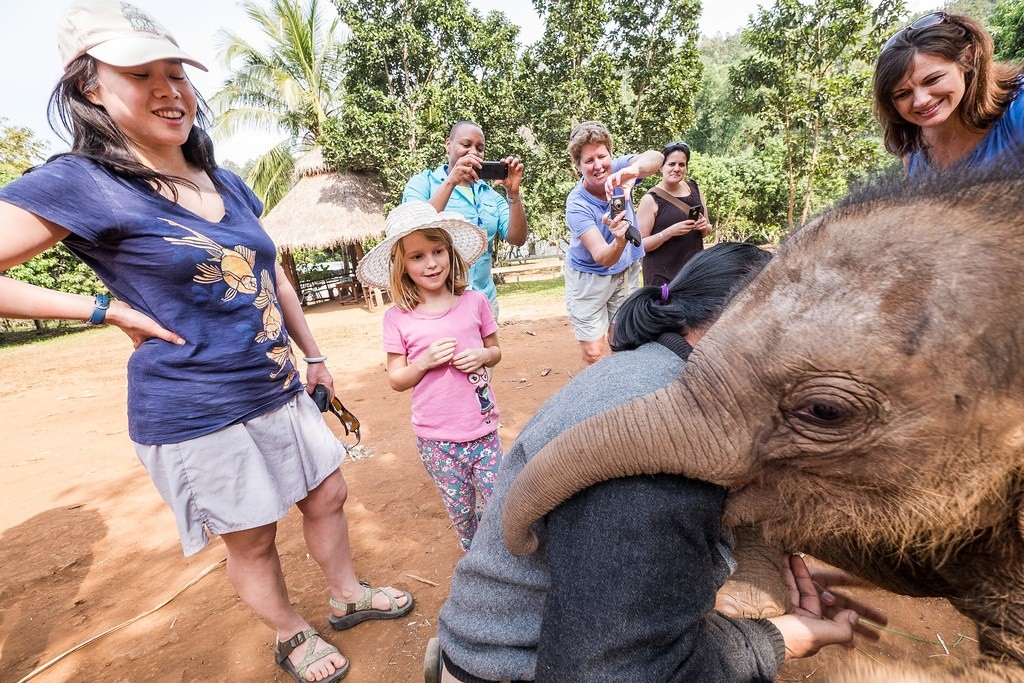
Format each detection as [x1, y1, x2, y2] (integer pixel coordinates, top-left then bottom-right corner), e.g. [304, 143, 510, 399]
[337, 282, 361, 305]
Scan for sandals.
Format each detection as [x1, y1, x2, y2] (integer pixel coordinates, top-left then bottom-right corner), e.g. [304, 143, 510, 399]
[329, 579, 414, 630]
[273, 629, 351, 683]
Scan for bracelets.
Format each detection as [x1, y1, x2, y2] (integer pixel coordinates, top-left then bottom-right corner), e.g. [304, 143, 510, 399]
[506, 191, 522, 203]
[82, 293, 110, 328]
[302, 356, 328, 363]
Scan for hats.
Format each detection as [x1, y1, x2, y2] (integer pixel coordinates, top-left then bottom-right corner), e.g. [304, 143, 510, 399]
[56, 0, 208, 72]
[356, 201, 489, 291]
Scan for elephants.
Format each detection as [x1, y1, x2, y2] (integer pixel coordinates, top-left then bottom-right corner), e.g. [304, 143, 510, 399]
[502, 144, 1024, 683]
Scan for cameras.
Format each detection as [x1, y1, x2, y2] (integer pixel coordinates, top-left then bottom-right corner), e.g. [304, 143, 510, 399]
[610, 195, 625, 220]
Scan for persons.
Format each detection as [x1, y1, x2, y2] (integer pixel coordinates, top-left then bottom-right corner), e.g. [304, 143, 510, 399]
[871, 11, 1024, 197]
[636, 141, 712, 287]
[357, 200, 504, 553]
[564, 121, 665, 368]
[423, 241, 859, 683]
[402, 121, 528, 323]
[0, 0, 414, 683]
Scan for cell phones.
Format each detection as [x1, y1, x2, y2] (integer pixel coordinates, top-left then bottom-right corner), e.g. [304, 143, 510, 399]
[688, 205, 701, 224]
[472, 162, 509, 180]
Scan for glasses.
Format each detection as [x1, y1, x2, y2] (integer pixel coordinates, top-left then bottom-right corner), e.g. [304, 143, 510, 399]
[665, 141, 690, 149]
[327, 396, 361, 450]
[876, 11, 946, 53]
